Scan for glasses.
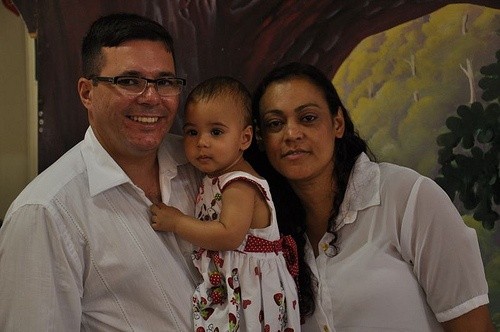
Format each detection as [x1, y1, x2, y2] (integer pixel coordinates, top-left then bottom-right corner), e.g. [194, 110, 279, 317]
[80, 71, 187, 99]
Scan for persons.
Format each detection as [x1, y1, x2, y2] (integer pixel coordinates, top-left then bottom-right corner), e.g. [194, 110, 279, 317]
[251, 60, 494, 332]
[150, 74, 302, 331]
[0, 13, 206, 332]
